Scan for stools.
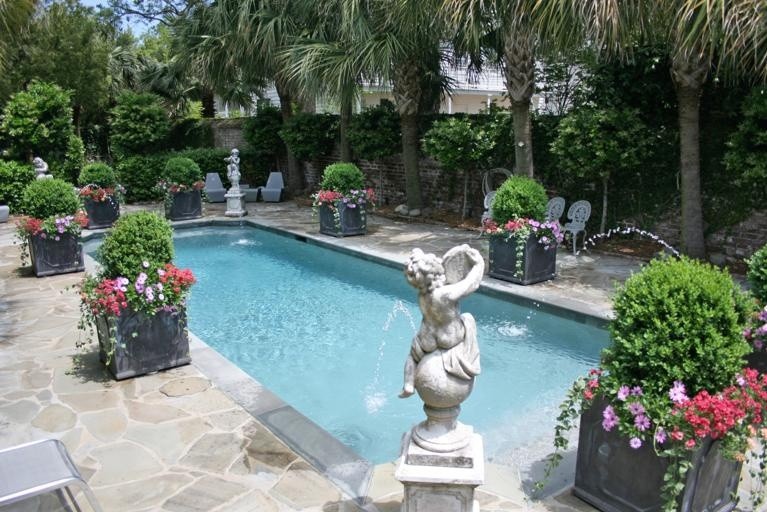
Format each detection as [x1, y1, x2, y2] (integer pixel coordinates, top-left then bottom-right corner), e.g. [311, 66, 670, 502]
[0, 437, 102, 512]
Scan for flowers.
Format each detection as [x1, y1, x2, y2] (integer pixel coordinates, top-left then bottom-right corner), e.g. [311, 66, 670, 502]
[10, 210, 88, 262]
[305, 184, 378, 231]
[51, 264, 198, 373]
[525, 357, 766, 512]
[156, 179, 205, 219]
[69, 184, 130, 207]
[479, 215, 567, 286]
[739, 308, 767, 353]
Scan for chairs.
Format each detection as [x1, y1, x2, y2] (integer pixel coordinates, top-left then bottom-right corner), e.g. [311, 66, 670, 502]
[204, 171, 226, 204]
[260, 170, 285, 203]
[545, 196, 564, 227]
[478, 190, 498, 238]
[559, 199, 592, 256]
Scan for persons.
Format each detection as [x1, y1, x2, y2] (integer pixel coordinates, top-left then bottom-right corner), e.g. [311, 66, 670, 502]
[223, 148, 241, 177]
[32, 157, 54, 181]
[396, 246, 484, 400]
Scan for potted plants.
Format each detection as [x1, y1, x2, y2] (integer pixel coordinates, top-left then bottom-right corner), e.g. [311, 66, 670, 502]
[74, 163, 123, 231]
[73, 207, 192, 379]
[568, 247, 748, 512]
[486, 177, 555, 286]
[0, 159, 14, 224]
[314, 160, 368, 237]
[163, 156, 203, 223]
[13, 179, 90, 278]
[733, 240, 766, 380]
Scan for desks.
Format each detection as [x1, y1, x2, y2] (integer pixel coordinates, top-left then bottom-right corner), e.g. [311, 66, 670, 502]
[239, 185, 259, 201]
